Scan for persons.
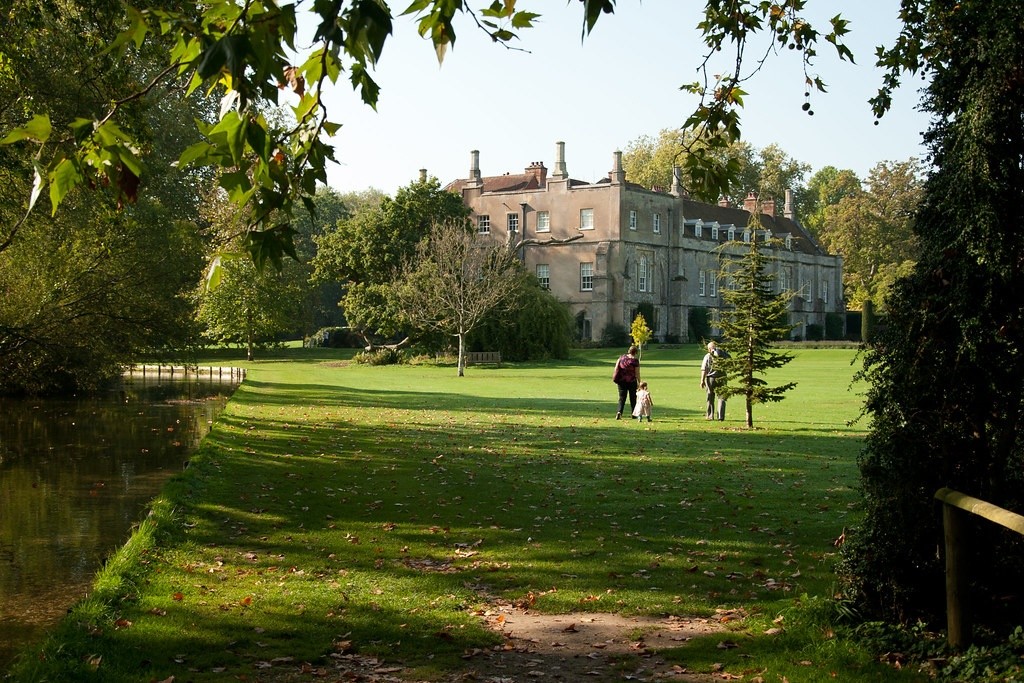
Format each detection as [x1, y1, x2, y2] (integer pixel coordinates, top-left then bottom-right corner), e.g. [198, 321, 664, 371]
[700, 342, 733, 421]
[633, 383, 653, 423]
[612, 346, 641, 420]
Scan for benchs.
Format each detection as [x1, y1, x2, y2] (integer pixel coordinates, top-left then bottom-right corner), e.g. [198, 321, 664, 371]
[464, 351, 501, 367]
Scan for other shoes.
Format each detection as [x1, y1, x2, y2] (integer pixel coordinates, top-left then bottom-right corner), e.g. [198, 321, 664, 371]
[638, 419, 642, 422]
[717, 418, 723, 421]
[705, 418, 713, 421]
[631, 413, 637, 419]
[647, 418, 652, 422]
[616, 412, 621, 420]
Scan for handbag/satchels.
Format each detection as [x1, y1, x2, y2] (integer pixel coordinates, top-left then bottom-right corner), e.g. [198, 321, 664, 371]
[613, 365, 623, 384]
